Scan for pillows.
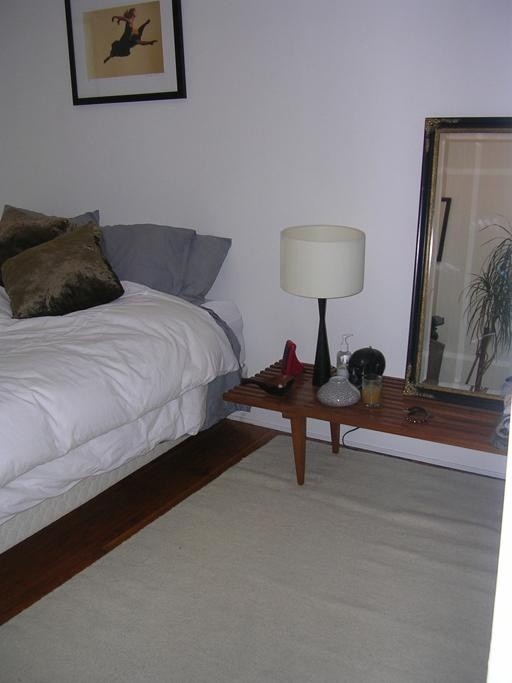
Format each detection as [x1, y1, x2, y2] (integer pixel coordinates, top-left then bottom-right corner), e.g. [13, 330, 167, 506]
[0, 204, 232, 321]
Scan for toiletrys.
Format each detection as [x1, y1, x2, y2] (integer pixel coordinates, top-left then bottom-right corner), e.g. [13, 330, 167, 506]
[337, 334, 353, 378]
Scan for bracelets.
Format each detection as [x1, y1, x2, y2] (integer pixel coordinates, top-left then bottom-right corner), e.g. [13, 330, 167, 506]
[403, 405, 431, 425]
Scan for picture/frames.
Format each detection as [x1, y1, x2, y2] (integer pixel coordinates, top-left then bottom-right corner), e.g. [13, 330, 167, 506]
[435, 194, 451, 262]
[62, 1, 188, 107]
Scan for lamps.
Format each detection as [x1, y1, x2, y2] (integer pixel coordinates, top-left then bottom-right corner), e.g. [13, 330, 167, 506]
[279, 223, 365, 388]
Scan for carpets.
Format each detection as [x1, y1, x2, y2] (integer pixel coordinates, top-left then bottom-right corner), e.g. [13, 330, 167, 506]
[0, 435, 506, 683]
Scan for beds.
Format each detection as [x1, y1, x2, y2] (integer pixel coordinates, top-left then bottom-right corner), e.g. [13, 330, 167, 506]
[1, 278, 251, 556]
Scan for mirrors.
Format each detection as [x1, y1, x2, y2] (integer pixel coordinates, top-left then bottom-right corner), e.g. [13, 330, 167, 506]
[401, 115, 512, 413]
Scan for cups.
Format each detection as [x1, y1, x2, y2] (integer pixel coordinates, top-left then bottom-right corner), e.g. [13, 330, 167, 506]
[361, 373, 384, 409]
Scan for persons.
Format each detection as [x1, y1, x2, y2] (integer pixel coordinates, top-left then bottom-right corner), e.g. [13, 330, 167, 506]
[103, 6, 159, 64]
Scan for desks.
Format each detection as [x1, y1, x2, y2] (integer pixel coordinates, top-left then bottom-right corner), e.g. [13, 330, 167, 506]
[221, 357, 509, 484]
